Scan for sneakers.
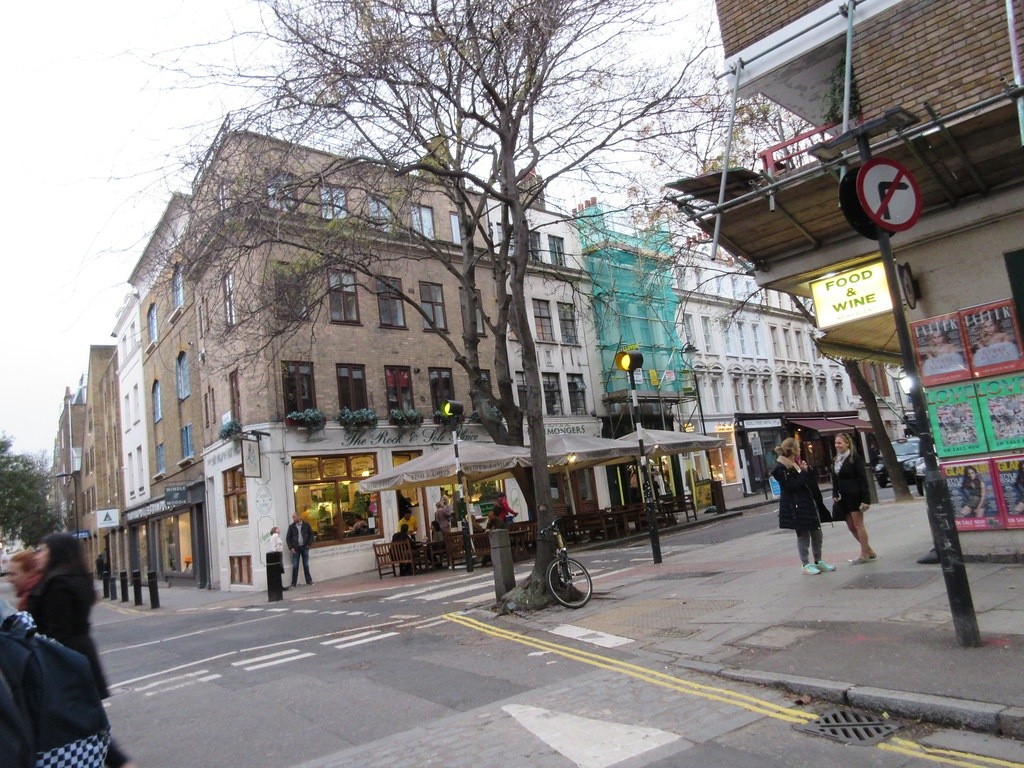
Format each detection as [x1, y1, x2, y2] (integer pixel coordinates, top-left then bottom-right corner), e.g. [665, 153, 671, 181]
[802, 563, 821, 575]
[813, 560, 836, 572]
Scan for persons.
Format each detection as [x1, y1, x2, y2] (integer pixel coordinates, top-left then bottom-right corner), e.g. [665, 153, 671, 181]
[770, 437, 837, 575]
[1014, 459, 1024, 514]
[831, 432, 877, 564]
[334, 487, 520, 577]
[630, 468, 640, 503]
[270, 526, 286, 590]
[287, 514, 316, 589]
[95, 554, 105, 577]
[959, 465, 986, 518]
[926, 324, 955, 359]
[976, 316, 1011, 348]
[9, 532, 136, 768]
[641, 465, 667, 506]
[0, 593, 111, 768]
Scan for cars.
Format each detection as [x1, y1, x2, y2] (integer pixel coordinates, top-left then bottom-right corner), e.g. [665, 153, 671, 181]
[914, 445, 942, 494]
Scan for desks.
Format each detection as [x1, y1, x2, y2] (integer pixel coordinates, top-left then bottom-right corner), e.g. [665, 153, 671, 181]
[502, 529, 532, 562]
[607, 508, 645, 536]
[415, 540, 446, 570]
[643, 501, 678, 525]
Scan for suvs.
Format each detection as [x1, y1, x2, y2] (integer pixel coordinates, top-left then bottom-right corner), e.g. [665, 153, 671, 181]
[873, 437, 924, 488]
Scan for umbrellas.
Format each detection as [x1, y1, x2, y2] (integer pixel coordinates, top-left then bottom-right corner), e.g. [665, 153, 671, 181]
[615, 428, 726, 504]
[359, 437, 567, 549]
[547, 432, 657, 535]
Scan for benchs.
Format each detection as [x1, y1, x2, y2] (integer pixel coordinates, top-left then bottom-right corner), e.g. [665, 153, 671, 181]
[666, 494, 697, 522]
[435, 531, 465, 572]
[508, 519, 539, 547]
[563, 513, 620, 542]
[467, 530, 491, 559]
[371, 541, 428, 577]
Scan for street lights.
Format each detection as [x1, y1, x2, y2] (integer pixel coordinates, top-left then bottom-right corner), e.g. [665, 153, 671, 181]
[680, 341, 729, 513]
[55, 471, 78, 542]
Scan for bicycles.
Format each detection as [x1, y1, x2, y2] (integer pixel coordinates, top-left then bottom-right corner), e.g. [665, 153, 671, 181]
[540, 515, 602, 610]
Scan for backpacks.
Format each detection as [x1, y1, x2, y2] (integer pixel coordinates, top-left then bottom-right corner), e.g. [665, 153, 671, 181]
[1, 613, 111, 768]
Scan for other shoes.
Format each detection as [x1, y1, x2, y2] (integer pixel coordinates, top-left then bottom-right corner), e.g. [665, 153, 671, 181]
[847, 555, 866, 565]
[869, 552, 877, 559]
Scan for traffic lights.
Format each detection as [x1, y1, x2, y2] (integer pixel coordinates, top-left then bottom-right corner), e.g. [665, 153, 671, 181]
[440, 398, 465, 420]
[614, 349, 642, 373]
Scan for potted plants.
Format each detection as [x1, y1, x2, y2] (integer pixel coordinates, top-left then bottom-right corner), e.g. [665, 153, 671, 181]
[284, 410, 327, 431]
[337, 407, 377, 426]
[470, 409, 502, 424]
[432, 410, 467, 424]
[218, 421, 240, 439]
[388, 408, 425, 427]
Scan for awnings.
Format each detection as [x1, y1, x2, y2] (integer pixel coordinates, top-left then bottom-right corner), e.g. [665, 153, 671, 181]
[785, 416, 856, 437]
[808, 260, 904, 368]
[826, 416, 873, 432]
